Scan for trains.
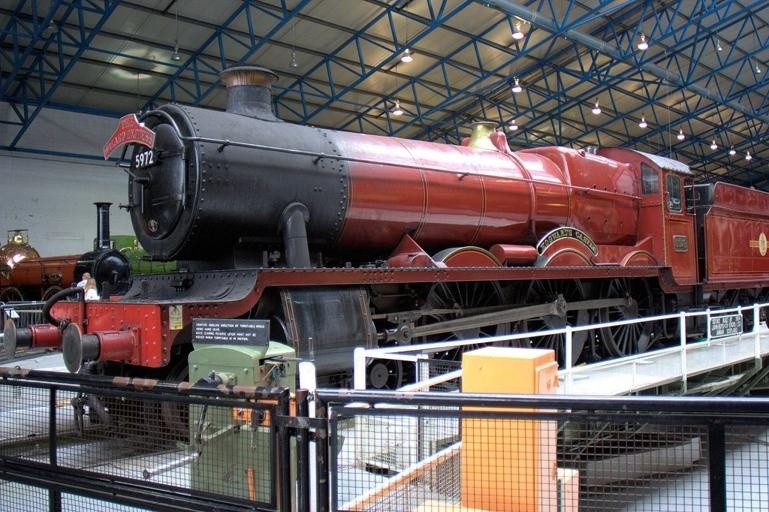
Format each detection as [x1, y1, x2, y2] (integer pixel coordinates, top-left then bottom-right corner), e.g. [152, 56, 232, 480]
[3, 66, 769, 440]
[0, 203, 130, 301]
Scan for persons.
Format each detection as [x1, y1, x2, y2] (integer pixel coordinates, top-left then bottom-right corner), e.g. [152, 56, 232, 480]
[76, 272, 91, 299]
[83, 279, 100, 300]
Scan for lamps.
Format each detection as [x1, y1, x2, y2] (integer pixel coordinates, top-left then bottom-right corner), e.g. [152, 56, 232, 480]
[714, 30, 761, 74]
[170, 0, 413, 118]
